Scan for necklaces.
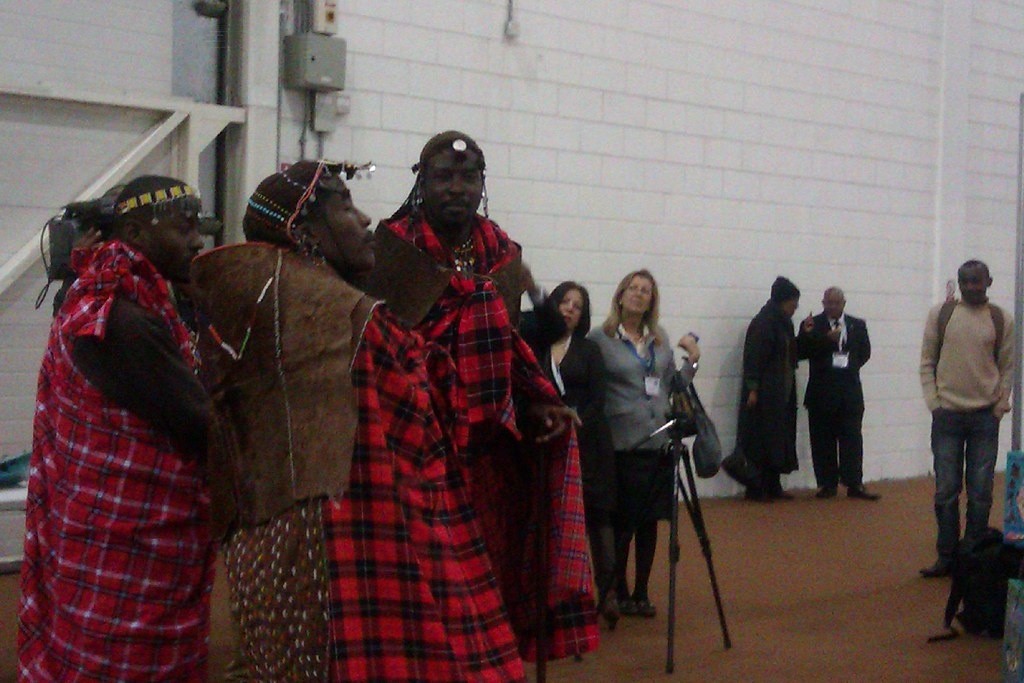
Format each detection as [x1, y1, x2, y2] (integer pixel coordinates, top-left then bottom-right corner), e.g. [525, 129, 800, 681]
[453, 236, 474, 278]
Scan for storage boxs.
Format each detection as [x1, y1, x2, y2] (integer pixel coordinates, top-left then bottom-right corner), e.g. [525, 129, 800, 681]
[1002, 578, 1024, 683]
[1001, 449, 1024, 547]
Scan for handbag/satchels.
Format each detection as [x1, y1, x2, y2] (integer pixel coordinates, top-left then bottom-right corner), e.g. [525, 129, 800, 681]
[720, 403, 763, 491]
[677, 370, 723, 479]
[669, 370, 698, 438]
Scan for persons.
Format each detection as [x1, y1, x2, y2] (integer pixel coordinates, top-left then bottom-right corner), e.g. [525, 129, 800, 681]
[546, 282, 620, 631]
[373, 132, 602, 683]
[804, 287, 882, 502]
[18, 176, 228, 683]
[737, 276, 814, 504]
[597, 268, 701, 618]
[920, 260, 1016, 579]
[190, 160, 457, 683]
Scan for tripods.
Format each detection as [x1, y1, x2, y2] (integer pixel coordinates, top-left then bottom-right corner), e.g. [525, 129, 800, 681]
[575, 439, 731, 674]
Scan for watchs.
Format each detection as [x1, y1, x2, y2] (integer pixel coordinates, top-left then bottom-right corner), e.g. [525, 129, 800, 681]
[683, 356, 698, 369]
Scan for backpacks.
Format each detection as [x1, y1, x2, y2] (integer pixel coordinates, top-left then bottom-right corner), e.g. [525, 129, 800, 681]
[931, 527, 1024, 639]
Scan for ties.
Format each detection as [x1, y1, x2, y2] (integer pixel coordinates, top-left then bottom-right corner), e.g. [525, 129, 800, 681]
[835, 320, 841, 352]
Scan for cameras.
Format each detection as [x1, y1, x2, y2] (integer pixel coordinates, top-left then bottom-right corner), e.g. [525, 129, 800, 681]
[664, 412, 696, 438]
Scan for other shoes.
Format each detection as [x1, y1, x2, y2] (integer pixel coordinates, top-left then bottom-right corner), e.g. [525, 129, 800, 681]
[769, 490, 795, 503]
[632, 594, 656, 618]
[921, 555, 955, 579]
[847, 485, 881, 500]
[608, 592, 638, 615]
[601, 599, 620, 631]
[817, 484, 837, 497]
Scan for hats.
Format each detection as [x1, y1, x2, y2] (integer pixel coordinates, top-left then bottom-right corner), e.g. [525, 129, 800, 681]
[771, 275, 800, 303]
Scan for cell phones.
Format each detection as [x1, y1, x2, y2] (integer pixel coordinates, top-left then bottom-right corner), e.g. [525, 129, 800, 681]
[679, 331, 699, 350]
